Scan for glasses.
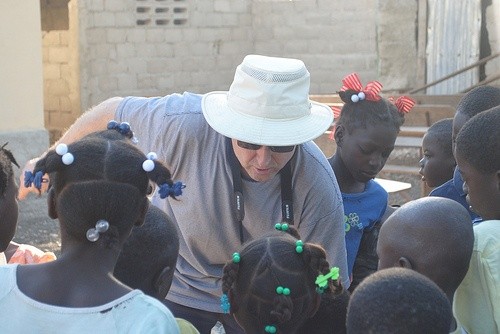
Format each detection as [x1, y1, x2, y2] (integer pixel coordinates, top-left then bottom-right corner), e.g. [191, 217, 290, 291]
[237, 140, 294, 153]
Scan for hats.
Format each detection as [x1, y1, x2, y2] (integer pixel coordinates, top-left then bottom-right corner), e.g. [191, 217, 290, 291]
[201, 54, 334, 146]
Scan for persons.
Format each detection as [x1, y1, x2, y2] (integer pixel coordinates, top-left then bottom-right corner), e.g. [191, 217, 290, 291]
[428, 85, 500, 224]
[113, 203, 201, 334]
[419, 118, 457, 188]
[0, 142, 57, 264]
[452, 105, 500, 334]
[327, 72, 416, 293]
[0, 120, 186, 334]
[220, 197, 475, 334]
[17, 54, 351, 334]
